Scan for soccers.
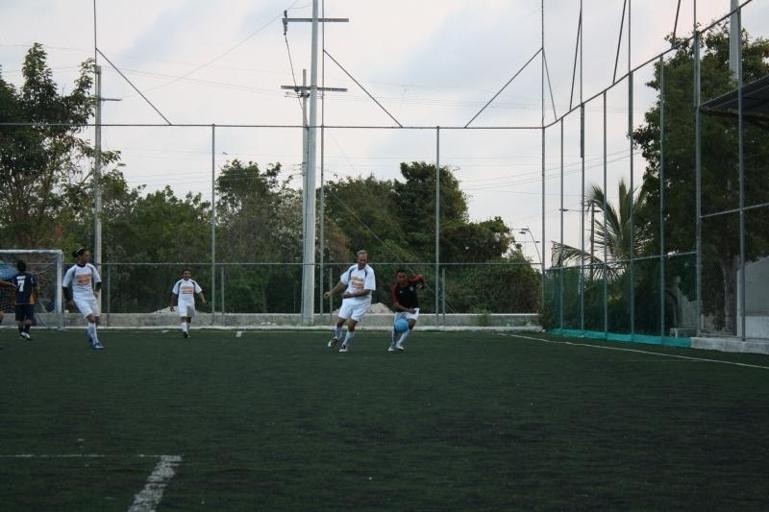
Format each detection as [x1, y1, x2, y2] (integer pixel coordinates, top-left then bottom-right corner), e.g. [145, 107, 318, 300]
[395, 319, 408, 333]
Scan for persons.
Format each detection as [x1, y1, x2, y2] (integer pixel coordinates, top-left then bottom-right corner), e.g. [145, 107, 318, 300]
[12, 262, 38, 340]
[170, 268, 206, 338]
[387, 271, 425, 352]
[62, 248, 104, 349]
[324, 250, 376, 352]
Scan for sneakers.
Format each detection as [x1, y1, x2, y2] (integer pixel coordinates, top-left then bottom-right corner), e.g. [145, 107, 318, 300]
[19, 327, 32, 341]
[183, 331, 192, 337]
[328, 337, 344, 348]
[339, 343, 353, 352]
[86, 330, 104, 352]
[388, 342, 404, 352]
